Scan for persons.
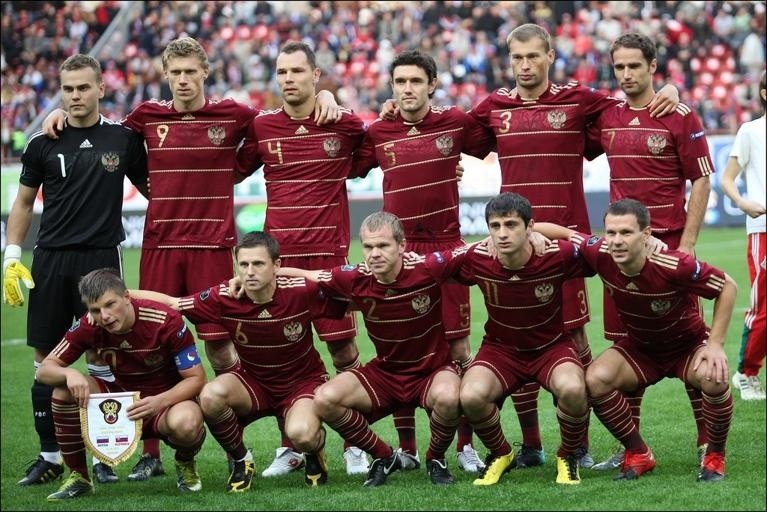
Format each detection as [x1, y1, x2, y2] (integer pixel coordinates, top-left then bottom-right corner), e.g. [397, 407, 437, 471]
[226, 210, 554, 490]
[34, 265, 207, 501]
[362, 191, 669, 487]
[480, 198, 740, 486]
[341, 49, 496, 473]
[0, 53, 148, 486]
[40, 36, 343, 483]
[82, 230, 424, 492]
[377, 21, 681, 473]
[578, 32, 716, 470]
[1, 0, 767, 162]
[717, 69, 766, 403]
[145, 38, 465, 479]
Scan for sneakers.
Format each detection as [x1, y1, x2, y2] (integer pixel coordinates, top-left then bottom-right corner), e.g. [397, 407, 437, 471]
[47, 471, 94, 501]
[93, 463, 120, 484]
[456, 444, 486, 475]
[613, 448, 654, 481]
[18, 455, 64, 486]
[590, 448, 625, 470]
[511, 442, 546, 468]
[363, 447, 402, 486]
[262, 446, 306, 477]
[397, 447, 421, 470]
[697, 444, 725, 484]
[578, 448, 594, 469]
[555, 455, 581, 485]
[426, 457, 453, 485]
[225, 460, 255, 493]
[175, 460, 202, 492]
[733, 371, 766, 401]
[344, 447, 370, 474]
[473, 449, 517, 486]
[305, 427, 327, 486]
[127, 453, 163, 481]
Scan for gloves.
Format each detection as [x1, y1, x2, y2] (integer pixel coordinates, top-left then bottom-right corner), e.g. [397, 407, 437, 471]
[3, 245, 35, 308]
